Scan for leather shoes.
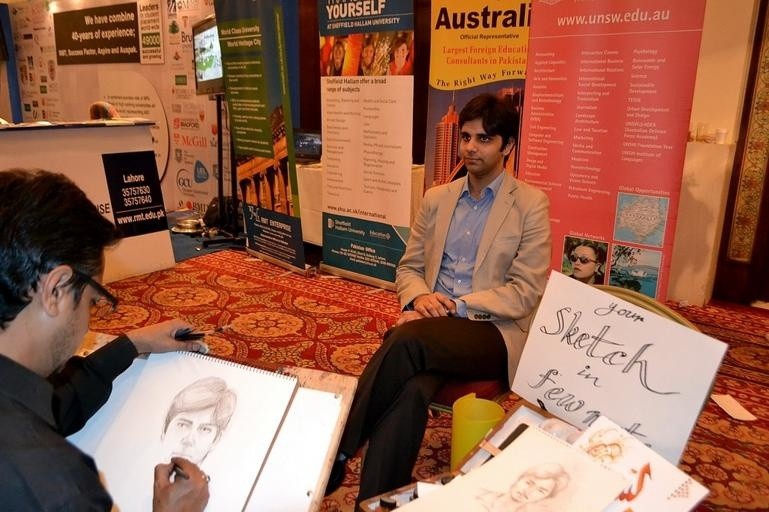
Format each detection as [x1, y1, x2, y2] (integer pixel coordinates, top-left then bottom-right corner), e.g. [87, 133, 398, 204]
[325, 457, 345, 497]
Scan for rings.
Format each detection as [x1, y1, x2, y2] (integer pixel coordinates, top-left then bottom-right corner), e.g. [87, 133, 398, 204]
[206, 476, 210, 483]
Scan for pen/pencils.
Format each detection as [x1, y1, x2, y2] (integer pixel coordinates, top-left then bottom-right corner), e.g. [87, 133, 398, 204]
[175, 324, 230, 341]
[173, 465, 189, 479]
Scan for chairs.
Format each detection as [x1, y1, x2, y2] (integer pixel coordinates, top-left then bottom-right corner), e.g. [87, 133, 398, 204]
[428, 378, 509, 417]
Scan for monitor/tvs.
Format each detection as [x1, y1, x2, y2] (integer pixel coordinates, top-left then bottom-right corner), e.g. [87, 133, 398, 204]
[192, 13, 225, 97]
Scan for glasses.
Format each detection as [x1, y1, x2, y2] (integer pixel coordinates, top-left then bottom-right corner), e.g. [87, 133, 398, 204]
[77, 273, 119, 318]
[571, 254, 597, 264]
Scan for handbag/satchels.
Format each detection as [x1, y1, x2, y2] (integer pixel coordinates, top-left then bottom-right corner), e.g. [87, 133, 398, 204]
[202, 196, 238, 229]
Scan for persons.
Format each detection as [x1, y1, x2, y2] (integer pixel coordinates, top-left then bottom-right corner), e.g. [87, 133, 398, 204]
[566, 241, 603, 285]
[0, 164, 210, 512]
[322, 92, 552, 512]
[158, 377, 237, 467]
[477, 458, 571, 512]
